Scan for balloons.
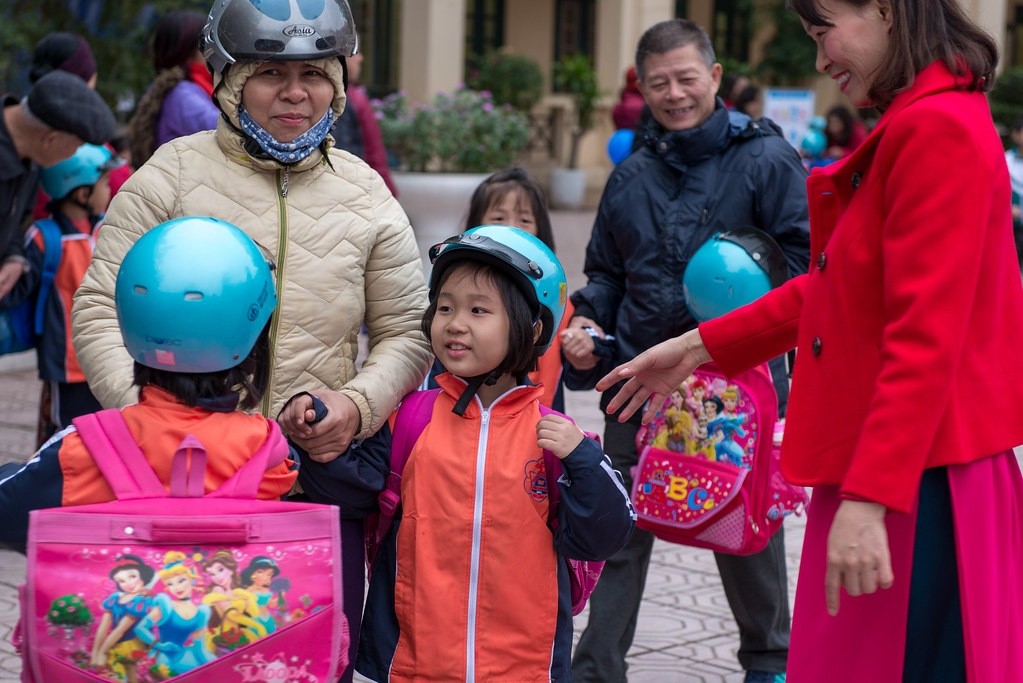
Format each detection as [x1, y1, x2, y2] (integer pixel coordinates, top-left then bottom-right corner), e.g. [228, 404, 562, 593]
[609, 93, 647, 164]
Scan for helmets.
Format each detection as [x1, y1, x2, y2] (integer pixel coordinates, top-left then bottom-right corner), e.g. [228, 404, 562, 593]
[200, 0, 359, 76]
[428, 225, 567, 357]
[35, 142, 114, 198]
[607, 128, 636, 162]
[682, 231, 798, 323]
[115, 216, 277, 373]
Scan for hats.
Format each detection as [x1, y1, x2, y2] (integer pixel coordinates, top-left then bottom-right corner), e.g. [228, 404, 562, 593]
[26, 31, 97, 83]
[28, 76, 118, 145]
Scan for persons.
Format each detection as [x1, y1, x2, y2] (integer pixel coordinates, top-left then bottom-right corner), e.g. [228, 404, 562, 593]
[597, 0, 1023, 683]
[70, 0, 435, 683]
[328, 51, 397, 199]
[0, 217, 299, 556]
[24, 142, 127, 446]
[569, 15, 812, 683]
[29, 34, 133, 216]
[1004, 116, 1023, 268]
[721, 73, 765, 120]
[465, 168, 600, 415]
[129, 9, 222, 171]
[0, 73, 118, 350]
[799, 107, 866, 168]
[276, 223, 637, 683]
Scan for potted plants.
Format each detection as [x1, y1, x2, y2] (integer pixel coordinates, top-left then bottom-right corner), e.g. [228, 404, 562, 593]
[552, 58, 598, 209]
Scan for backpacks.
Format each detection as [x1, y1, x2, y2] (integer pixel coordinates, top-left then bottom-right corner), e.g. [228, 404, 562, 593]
[11, 405, 349, 683]
[375, 393, 611, 615]
[630, 350, 812, 555]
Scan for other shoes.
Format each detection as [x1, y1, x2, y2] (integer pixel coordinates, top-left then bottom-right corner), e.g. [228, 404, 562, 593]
[742, 670, 788, 683]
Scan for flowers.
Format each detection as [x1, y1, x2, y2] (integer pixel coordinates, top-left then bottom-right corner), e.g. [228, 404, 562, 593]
[368, 82, 527, 175]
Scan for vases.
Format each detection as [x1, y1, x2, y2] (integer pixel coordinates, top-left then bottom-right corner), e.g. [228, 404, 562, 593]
[392, 171, 508, 244]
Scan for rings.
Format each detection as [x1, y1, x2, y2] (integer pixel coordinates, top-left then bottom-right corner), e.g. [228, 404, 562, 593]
[567, 332, 573, 340]
